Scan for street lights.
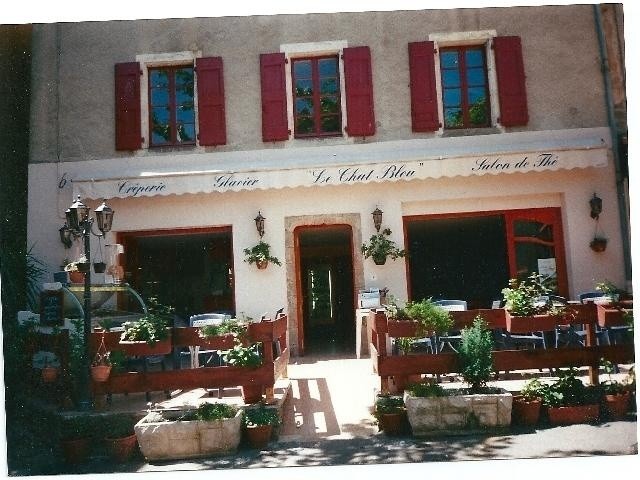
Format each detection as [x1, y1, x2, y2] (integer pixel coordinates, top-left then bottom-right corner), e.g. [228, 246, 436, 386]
[59, 194, 114, 412]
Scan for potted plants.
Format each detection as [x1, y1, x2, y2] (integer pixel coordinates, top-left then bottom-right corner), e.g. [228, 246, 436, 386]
[54, 415, 91, 465]
[88, 352, 114, 386]
[194, 311, 254, 351]
[511, 369, 542, 424]
[112, 306, 172, 356]
[359, 234, 411, 268]
[239, 239, 282, 271]
[371, 390, 406, 436]
[593, 379, 633, 419]
[241, 401, 281, 446]
[62, 255, 90, 288]
[589, 219, 609, 254]
[536, 372, 602, 425]
[375, 287, 457, 349]
[500, 269, 560, 337]
[42, 362, 68, 383]
[228, 337, 267, 372]
[102, 412, 138, 461]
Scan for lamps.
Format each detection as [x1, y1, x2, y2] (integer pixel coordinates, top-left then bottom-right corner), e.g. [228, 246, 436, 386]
[372, 207, 384, 232]
[588, 190, 602, 220]
[254, 210, 266, 239]
